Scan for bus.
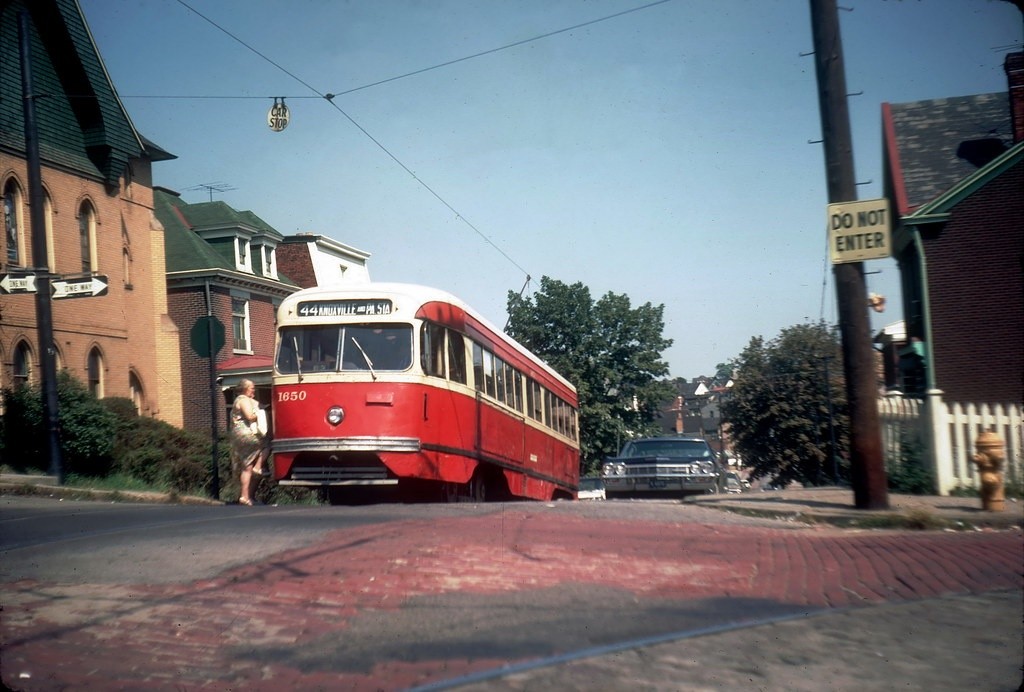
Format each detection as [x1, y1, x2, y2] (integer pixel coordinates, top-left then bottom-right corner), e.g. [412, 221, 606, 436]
[266, 279, 581, 505]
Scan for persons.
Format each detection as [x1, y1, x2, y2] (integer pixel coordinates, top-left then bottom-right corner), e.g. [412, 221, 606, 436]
[393, 342, 411, 369]
[230, 378, 272, 506]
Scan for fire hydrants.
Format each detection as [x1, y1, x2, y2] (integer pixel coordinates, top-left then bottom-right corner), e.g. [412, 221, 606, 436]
[968, 429, 1008, 511]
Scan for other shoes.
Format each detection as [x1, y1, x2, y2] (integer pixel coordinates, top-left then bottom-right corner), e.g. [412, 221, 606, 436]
[252, 468, 264, 476]
[238, 497, 253, 507]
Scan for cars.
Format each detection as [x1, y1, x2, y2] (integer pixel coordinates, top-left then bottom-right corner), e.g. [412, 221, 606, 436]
[601, 435, 751, 499]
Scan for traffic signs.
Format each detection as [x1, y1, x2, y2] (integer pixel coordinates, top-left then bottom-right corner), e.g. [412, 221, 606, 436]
[0, 268, 38, 295]
[51, 274, 109, 300]
